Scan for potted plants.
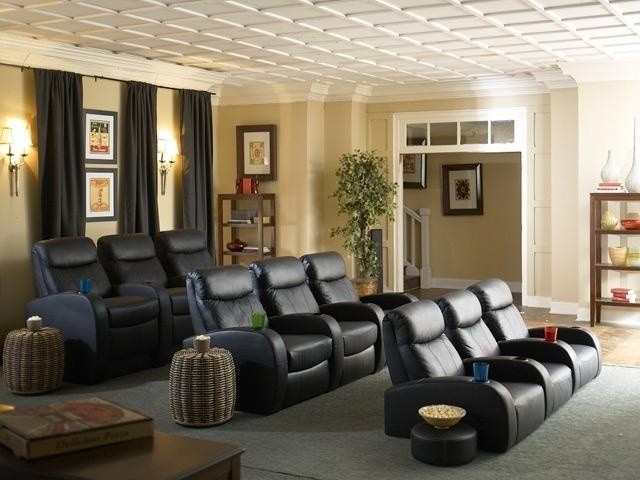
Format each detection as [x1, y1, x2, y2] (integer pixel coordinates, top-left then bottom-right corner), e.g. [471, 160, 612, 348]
[328, 150, 401, 298]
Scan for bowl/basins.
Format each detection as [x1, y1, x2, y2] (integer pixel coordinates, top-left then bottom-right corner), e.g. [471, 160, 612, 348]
[620, 220, 640, 231]
[418, 404, 467, 429]
[629, 253, 640, 267]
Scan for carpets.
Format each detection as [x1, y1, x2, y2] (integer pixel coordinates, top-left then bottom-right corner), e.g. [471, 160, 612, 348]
[0, 344, 639, 480]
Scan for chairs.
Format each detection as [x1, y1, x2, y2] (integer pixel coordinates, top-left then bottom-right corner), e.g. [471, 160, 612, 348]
[182, 251, 418, 416]
[383, 278, 602, 453]
[26, 229, 218, 384]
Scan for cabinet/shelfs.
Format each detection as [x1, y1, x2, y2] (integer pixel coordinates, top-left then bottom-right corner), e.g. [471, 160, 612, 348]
[0, 430, 242, 480]
[590, 193, 640, 327]
[217, 194, 275, 267]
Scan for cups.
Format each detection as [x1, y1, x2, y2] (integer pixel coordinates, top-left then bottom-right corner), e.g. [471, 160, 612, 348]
[544, 325, 558, 342]
[80, 278, 93, 294]
[473, 362, 490, 382]
[251, 313, 266, 332]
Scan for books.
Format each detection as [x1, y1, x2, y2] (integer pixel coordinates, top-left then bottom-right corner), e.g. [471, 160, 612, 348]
[242, 246, 271, 253]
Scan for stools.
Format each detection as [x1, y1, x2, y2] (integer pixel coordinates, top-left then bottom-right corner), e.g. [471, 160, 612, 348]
[4, 328, 65, 394]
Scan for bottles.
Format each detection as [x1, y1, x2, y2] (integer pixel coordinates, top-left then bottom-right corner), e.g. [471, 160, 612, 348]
[602, 211, 618, 230]
[600, 149, 622, 184]
[225, 239, 246, 252]
[625, 117, 639, 194]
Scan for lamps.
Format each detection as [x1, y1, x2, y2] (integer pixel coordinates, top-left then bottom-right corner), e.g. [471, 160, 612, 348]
[157, 125, 180, 197]
[0, 112, 34, 197]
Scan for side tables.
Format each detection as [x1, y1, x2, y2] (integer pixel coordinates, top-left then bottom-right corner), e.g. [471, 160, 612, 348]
[168, 347, 237, 426]
[410, 420, 478, 469]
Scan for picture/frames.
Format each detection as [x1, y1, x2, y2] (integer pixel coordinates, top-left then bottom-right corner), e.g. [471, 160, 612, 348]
[235, 122, 278, 182]
[84, 109, 117, 164]
[83, 167, 118, 223]
[441, 162, 483, 218]
[404, 137, 428, 190]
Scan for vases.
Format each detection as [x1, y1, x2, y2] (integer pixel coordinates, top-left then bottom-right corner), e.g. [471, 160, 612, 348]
[600, 144, 619, 180]
[623, 118, 640, 193]
[600, 208, 616, 228]
